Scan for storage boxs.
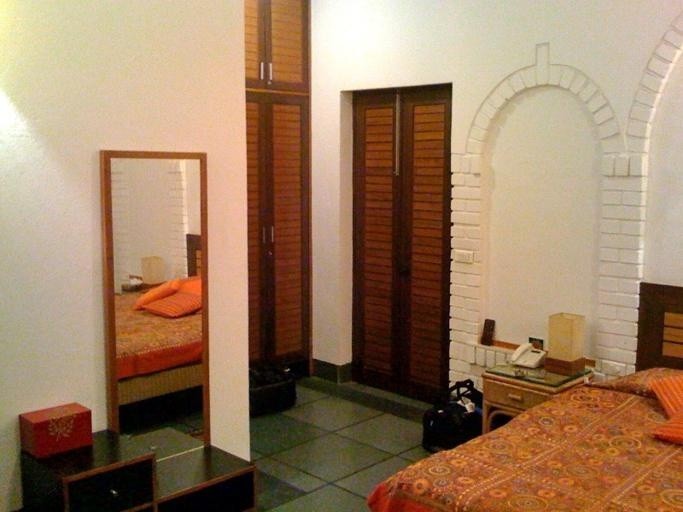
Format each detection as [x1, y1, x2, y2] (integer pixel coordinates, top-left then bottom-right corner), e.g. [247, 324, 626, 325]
[17, 402, 91, 459]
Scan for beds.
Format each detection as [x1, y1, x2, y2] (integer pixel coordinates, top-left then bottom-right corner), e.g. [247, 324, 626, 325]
[111, 233, 203, 407]
[365, 281, 682, 511]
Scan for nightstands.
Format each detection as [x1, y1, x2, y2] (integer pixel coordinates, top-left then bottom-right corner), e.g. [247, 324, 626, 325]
[480, 363, 594, 437]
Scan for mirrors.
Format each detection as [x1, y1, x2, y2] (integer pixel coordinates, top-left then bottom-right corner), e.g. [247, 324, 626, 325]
[97, 151, 208, 447]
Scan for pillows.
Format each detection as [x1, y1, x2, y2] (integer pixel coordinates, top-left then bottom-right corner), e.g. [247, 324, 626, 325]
[132, 279, 202, 320]
[606, 365, 682, 447]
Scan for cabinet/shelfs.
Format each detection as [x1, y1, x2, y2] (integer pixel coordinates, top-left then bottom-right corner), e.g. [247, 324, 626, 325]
[21, 429, 257, 510]
[351, 84, 448, 408]
[243, 2, 309, 420]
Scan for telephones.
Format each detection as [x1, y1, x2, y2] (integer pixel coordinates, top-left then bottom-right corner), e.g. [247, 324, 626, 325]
[511, 342, 547, 369]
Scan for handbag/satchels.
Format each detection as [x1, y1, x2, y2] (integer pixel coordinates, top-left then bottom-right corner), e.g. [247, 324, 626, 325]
[421, 379, 483, 454]
